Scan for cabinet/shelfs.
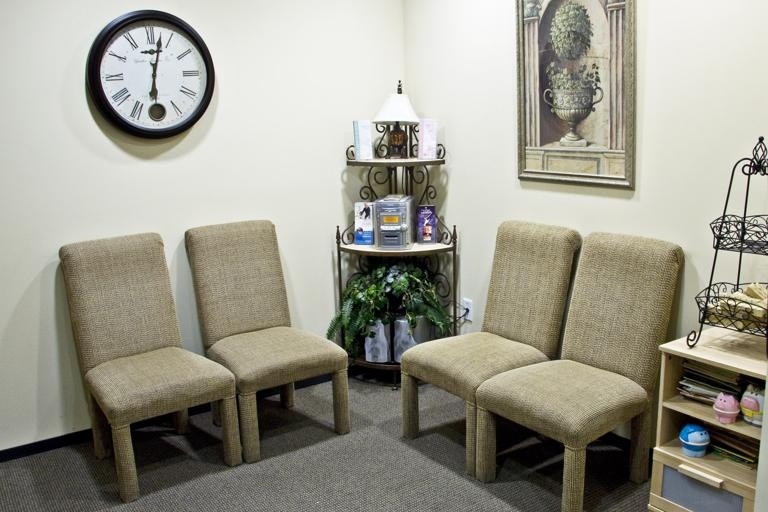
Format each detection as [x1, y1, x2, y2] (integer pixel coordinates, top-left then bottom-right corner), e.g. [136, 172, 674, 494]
[336, 81, 456, 387]
[647, 324, 768, 512]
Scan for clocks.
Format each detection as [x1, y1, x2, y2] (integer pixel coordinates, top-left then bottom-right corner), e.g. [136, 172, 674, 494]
[86, 10, 214, 138]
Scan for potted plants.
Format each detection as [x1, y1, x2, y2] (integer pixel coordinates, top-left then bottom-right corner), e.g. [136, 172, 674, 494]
[326, 262, 392, 364]
[385, 262, 451, 364]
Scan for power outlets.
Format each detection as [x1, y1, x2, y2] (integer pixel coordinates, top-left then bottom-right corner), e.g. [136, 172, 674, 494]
[462, 298, 473, 322]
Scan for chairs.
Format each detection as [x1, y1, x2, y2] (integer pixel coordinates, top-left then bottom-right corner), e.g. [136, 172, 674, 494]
[59, 233, 243, 504]
[475, 232, 685, 512]
[401, 220, 583, 477]
[184, 219, 350, 463]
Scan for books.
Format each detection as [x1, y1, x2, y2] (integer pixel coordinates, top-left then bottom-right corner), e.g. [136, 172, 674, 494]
[675, 358, 741, 406]
[703, 425, 760, 471]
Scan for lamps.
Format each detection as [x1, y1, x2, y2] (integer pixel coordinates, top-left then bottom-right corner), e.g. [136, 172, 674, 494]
[372, 96, 420, 159]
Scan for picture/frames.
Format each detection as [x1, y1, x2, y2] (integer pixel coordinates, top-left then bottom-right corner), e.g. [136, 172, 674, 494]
[517, 0, 635, 190]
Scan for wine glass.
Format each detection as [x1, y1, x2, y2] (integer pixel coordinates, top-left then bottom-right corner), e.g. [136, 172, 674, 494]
[544, 88, 605, 141]
